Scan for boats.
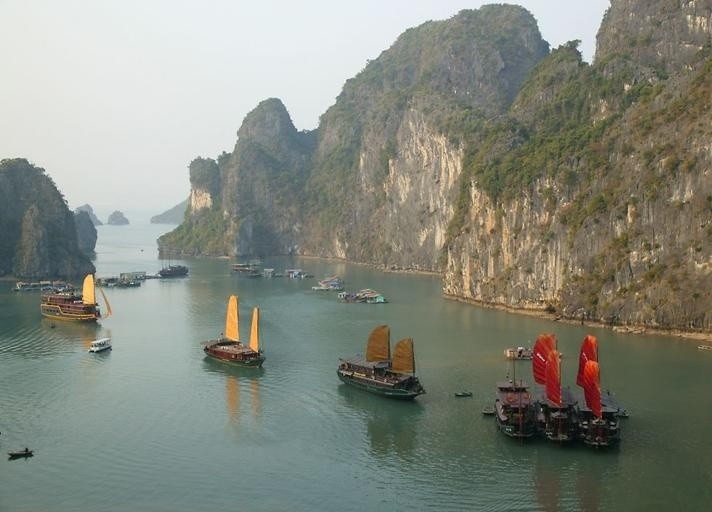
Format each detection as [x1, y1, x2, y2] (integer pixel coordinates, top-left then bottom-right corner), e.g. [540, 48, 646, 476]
[11, 261, 188, 321]
[88, 338, 112, 352]
[312, 276, 344, 291]
[232, 261, 313, 279]
[455, 389, 472, 396]
[336, 289, 385, 303]
[7, 448, 33, 456]
[504, 343, 534, 361]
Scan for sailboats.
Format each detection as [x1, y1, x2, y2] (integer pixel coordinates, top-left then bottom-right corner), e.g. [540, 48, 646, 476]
[200, 295, 266, 368]
[483, 334, 630, 449]
[336, 325, 426, 400]
